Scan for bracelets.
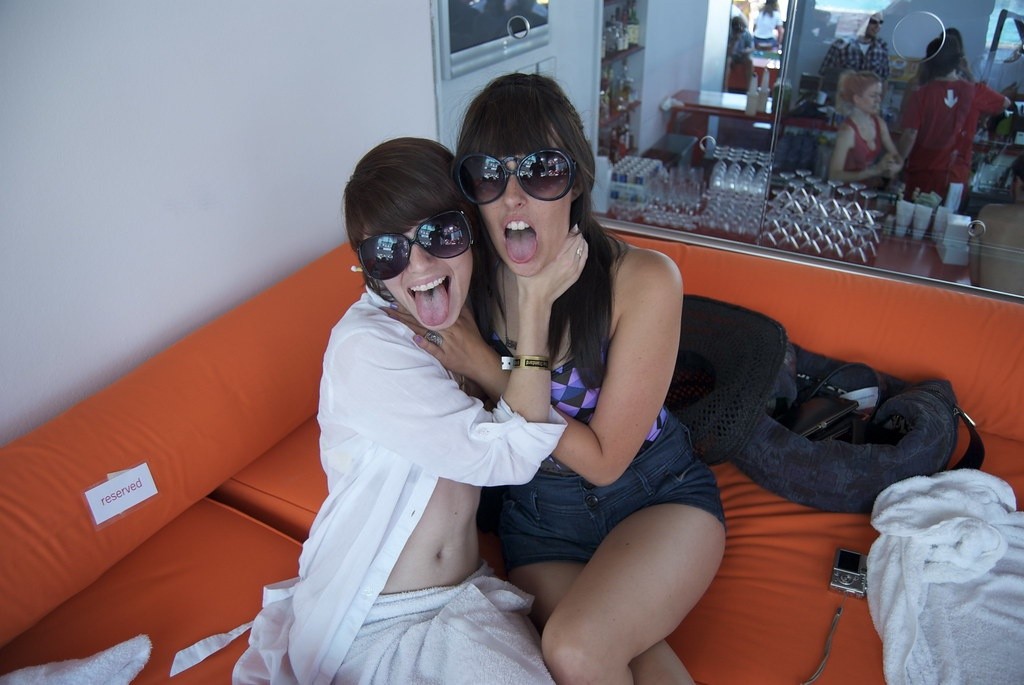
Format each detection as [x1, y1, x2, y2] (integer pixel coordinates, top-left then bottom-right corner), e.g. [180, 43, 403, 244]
[501, 355, 552, 371]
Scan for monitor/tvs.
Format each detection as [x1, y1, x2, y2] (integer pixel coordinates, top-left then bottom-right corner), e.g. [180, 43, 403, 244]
[438, 0, 549, 81]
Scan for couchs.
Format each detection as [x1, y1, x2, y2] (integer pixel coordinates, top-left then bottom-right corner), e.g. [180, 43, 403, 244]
[0, 232, 1023, 684]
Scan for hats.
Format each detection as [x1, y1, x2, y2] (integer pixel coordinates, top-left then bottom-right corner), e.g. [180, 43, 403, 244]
[663, 294, 786, 465]
[803, 362, 887, 425]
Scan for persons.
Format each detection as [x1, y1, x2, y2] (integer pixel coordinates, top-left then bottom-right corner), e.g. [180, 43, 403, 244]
[895, 28, 1011, 214]
[827, 67, 904, 208]
[817, 0, 890, 99]
[169, 136, 588, 685]
[724, 0, 784, 90]
[379, 72, 727, 685]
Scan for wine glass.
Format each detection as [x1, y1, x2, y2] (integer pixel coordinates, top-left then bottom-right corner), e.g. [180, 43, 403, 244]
[646, 146, 879, 264]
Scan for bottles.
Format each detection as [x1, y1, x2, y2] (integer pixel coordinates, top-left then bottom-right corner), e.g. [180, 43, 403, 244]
[598, 0, 637, 163]
[772, 79, 793, 115]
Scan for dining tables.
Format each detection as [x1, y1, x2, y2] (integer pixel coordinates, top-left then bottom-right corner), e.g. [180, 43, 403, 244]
[667, 88, 1023, 158]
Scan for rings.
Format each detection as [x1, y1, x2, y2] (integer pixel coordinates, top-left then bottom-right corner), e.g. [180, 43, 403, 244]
[424, 330, 443, 346]
[577, 246, 583, 257]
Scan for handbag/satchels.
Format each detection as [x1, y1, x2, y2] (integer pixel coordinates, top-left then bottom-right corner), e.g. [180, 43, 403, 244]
[730, 334, 959, 515]
[781, 393, 861, 441]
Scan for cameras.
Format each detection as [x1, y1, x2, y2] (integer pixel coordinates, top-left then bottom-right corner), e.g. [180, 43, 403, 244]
[828, 548, 868, 598]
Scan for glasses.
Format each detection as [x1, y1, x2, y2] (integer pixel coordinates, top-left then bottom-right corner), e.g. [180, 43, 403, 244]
[357, 210, 473, 280]
[457, 149, 578, 204]
[862, 18, 884, 25]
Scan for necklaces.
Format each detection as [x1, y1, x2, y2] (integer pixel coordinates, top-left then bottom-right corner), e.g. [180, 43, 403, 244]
[500, 263, 518, 350]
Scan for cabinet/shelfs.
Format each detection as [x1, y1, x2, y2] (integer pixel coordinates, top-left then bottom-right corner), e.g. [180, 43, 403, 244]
[599, 0, 645, 163]
